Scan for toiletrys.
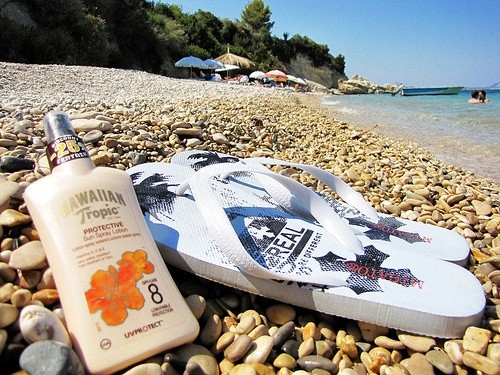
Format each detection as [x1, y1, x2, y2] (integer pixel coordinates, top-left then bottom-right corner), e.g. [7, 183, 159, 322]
[22, 110, 201, 375]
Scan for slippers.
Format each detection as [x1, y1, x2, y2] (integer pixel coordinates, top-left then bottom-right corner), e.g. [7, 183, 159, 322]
[123, 161, 486, 339]
[170, 150, 470, 269]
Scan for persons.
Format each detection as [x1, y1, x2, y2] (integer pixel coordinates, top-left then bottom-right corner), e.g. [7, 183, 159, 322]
[468, 90, 489, 104]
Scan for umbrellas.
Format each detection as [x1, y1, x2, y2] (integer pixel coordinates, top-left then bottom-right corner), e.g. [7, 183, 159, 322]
[294, 78, 307, 85]
[266, 69, 288, 82]
[202, 58, 223, 78]
[286, 74, 296, 81]
[215, 64, 240, 79]
[249, 71, 266, 78]
[175, 56, 208, 80]
